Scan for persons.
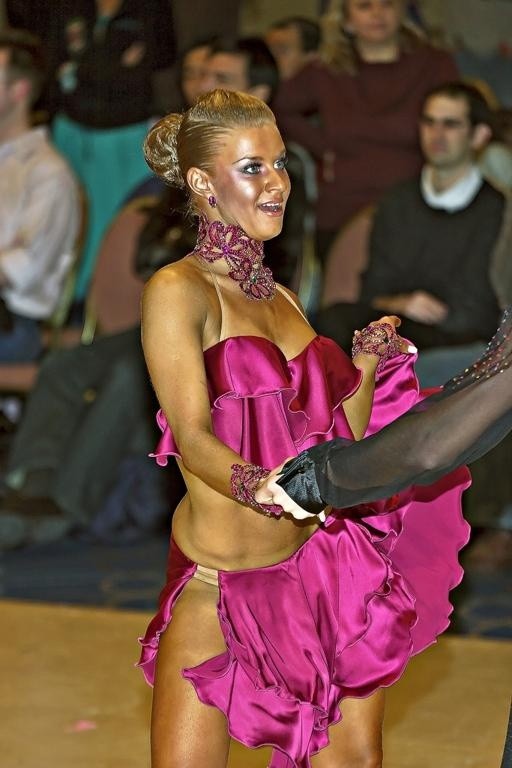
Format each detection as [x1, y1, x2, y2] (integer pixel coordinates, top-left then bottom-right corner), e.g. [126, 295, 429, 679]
[2, 0, 512, 552]
[253, 309, 512, 766]
[129, 88, 475, 766]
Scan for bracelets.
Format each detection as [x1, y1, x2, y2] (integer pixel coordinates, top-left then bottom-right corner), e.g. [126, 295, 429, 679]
[229, 462, 283, 519]
[352, 322, 401, 372]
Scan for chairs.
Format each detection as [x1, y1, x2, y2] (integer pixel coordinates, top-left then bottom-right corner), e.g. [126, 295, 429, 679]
[0, 60, 512, 363]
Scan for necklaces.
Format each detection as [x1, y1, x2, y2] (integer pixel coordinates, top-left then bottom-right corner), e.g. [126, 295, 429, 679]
[193, 212, 277, 303]
